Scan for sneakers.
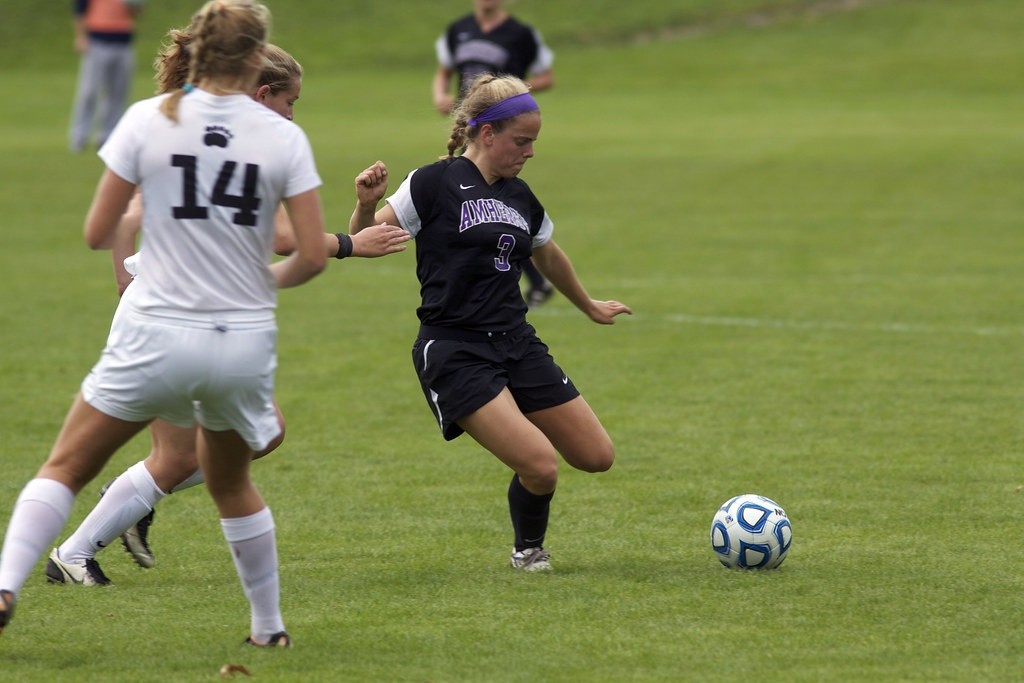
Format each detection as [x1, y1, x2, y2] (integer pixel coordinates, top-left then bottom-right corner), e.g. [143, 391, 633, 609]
[44, 547, 115, 588]
[509, 546, 554, 574]
[99, 477, 156, 569]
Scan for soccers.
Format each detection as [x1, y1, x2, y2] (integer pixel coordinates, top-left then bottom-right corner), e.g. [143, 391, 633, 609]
[710, 493, 793, 571]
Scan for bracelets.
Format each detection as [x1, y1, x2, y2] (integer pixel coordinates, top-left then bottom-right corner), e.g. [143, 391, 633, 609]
[332, 232, 353, 258]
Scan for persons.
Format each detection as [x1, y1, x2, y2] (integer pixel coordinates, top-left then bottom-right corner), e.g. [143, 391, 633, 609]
[1, 1, 328, 651]
[350, 74, 634, 576]
[430, 0, 555, 310]
[43, 40, 414, 584]
[70, 1, 143, 155]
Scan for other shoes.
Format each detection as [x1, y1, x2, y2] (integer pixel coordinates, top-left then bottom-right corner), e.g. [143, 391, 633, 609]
[0, 588, 17, 633]
[524, 282, 555, 307]
[243, 631, 292, 650]
